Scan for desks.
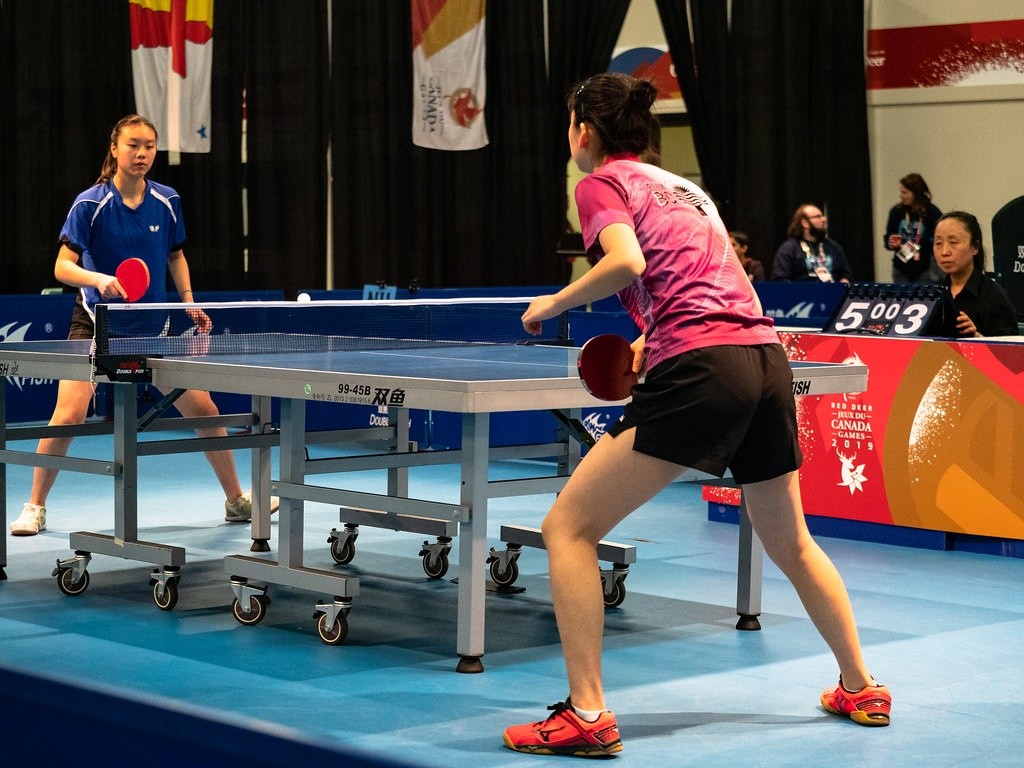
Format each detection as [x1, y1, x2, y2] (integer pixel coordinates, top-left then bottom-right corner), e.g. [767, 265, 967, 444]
[0, 333, 867, 676]
[702, 330, 1024, 558]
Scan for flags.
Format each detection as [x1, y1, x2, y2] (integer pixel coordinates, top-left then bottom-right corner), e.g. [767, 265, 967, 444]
[128, 0, 215, 153]
[411, 1, 489, 153]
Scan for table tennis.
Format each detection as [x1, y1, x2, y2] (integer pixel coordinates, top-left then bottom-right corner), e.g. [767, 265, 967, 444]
[297, 291, 312, 306]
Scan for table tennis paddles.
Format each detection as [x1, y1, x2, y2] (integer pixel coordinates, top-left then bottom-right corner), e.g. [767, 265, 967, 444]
[100, 258, 150, 304]
[578, 332, 639, 402]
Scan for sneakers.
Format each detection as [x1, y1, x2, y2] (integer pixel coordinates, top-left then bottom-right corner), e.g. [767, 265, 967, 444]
[9, 502, 48, 535]
[224, 489, 280, 522]
[821, 670, 893, 727]
[503, 695, 624, 757]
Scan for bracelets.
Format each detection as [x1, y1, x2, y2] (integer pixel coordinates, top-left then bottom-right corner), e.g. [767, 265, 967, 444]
[179, 290, 193, 298]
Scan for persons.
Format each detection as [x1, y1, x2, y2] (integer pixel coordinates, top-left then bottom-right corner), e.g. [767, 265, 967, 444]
[12, 116, 280, 536]
[773, 203, 853, 285]
[721, 231, 764, 280]
[883, 171, 945, 282]
[503, 74, 892, 757]
[928, 210, 1020, 339]
